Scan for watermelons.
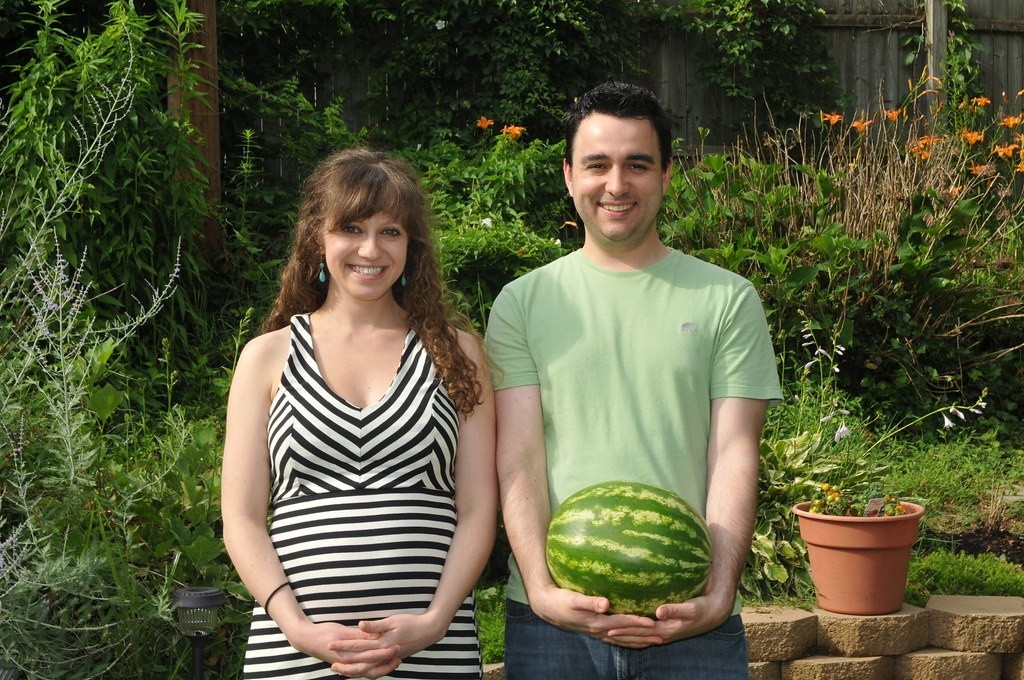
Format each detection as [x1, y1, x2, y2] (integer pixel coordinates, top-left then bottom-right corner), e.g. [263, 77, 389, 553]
[546, 482, 712, 616]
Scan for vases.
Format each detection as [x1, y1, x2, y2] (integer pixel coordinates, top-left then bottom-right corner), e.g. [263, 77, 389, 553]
[790, 501, 925, 617]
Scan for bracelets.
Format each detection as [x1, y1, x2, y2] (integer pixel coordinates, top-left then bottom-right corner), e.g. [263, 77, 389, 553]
[264, 582, 290, 617]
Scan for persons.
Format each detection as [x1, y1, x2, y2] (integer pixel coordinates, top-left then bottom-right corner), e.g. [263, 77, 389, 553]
[223, 146, 500, 680]
[484, 83, 784, 679]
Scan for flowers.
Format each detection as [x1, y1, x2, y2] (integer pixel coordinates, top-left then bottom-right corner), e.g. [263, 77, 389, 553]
[789, 304, 989, 516]
